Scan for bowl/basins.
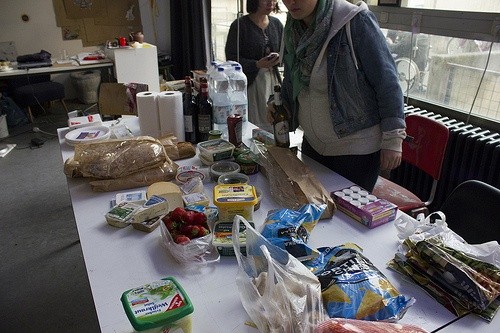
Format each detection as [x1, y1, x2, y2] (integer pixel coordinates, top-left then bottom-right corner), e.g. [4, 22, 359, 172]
[210, 159, 263, 212]
[175, 164, 206, 184]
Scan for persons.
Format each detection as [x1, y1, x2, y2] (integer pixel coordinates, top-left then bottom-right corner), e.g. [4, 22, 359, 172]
[267, 0, 406, 193]
[225, 0, 284, 133]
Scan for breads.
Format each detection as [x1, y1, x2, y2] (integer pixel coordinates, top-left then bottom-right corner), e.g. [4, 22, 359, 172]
[75, 141, 196, 191]
[130, 176, 210, 224]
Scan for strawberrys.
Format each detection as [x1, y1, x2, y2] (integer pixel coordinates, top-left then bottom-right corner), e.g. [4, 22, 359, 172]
[163, 208, 210, 245]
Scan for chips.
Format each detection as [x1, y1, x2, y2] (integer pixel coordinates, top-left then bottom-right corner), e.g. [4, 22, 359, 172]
[323, 278, 401, 318]
[302, 220, 317, 233]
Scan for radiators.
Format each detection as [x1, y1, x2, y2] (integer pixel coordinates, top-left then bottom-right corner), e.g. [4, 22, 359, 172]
[403, 102, 500, 212]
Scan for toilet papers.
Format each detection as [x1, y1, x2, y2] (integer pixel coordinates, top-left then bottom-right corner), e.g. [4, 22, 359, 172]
[136, 91, 160, 137]
[158, 90, 185, 142]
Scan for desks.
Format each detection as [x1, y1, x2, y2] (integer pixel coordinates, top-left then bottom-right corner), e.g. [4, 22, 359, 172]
[56, 118, 500, 333]
[1, 53, 113, 83]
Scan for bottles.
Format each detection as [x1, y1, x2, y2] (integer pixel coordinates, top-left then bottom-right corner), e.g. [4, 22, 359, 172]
[194, 77, 213, 143]
[205, 60, 249, 124]
[181, 75, 197, 145]
[272, 85, 290, 148]
[134, 32, 145, 43]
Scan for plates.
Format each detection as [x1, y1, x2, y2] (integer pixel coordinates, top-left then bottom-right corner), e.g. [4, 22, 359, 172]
[64, 126, 111, 147]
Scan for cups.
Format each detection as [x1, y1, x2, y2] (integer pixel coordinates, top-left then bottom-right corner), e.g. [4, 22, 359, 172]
[228, 114, 242, 146]
[120, 36, 126, 46]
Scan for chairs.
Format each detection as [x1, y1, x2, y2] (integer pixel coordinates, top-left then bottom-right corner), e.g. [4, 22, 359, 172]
[19, 51, 68, 121]
[98, 82, 148, 120]
[373, 112, 449, 209]
[432, 180, 500, 245]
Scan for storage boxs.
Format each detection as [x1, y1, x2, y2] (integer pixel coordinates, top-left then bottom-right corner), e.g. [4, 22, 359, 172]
[331, 184, 397, 229]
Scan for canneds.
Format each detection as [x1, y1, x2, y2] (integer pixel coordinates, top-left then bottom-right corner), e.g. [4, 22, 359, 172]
[208, 130, 223, 140]
[233, 146, 259, 175]
